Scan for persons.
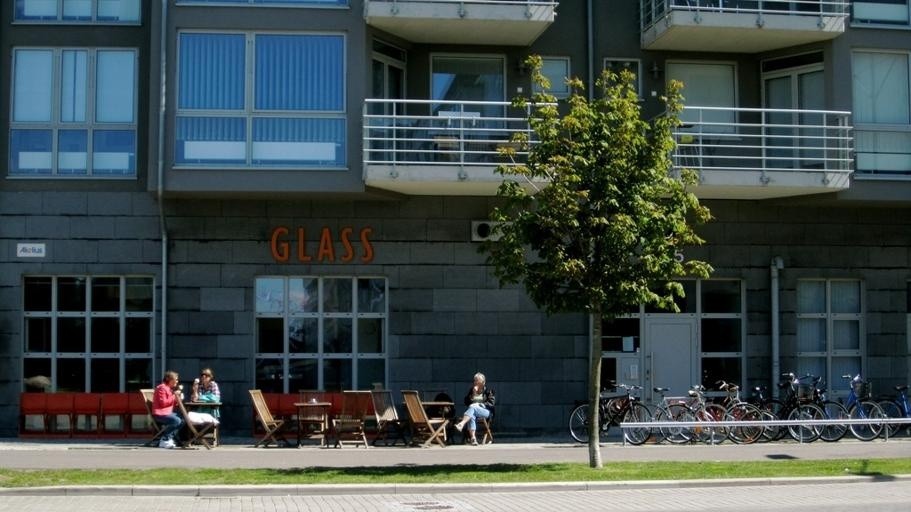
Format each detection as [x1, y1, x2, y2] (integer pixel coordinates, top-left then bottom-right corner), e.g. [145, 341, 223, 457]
[454, 372, 498, 447]
[190, 369, 222, 418]
[152, 370, 185, 449]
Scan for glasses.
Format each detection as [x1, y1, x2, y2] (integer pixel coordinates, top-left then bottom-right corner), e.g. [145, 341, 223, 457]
[201, 373, 210, 378]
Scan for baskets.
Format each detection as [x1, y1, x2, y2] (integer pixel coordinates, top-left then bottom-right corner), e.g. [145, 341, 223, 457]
[798, 382, 815, 402]
[853, 381, 873, 399]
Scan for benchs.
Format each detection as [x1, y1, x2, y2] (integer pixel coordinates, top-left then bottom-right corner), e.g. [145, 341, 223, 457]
[16, 392, 172, 441]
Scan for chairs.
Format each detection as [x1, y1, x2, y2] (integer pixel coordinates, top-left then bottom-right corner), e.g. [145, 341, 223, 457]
[247, 383, 455, 451]
[456, 393, 496, 447]
[138, 388, 184, 448]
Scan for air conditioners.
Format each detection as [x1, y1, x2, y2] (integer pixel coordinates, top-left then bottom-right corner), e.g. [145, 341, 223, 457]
[469, 220, 518, 244]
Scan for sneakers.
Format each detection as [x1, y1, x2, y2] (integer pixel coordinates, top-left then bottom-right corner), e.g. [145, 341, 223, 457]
[454, 423, 464, 433]
[159, 440, 176, 449]
[470, 439, 478, 446]
